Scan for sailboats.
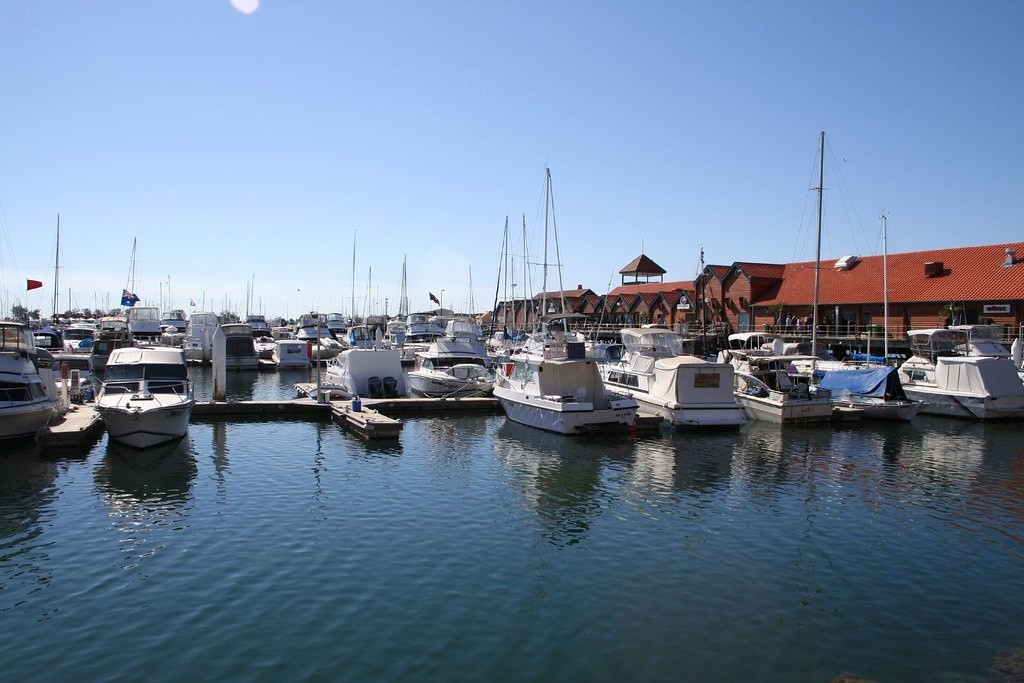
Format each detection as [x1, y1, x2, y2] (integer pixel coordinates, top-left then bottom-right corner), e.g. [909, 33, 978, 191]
[32, 167, 627, 364]
[808, 131, 929, 422]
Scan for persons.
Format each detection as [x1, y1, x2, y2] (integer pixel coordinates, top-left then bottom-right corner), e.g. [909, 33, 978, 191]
[944, 315, 960, 329]
[619, 319, 634, 328]
[656, 318, 702, 332]
[777, 314, 829, 334]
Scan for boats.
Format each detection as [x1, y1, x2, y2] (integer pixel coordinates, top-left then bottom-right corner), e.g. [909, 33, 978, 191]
[0, 322, 65, 439]
[93, 346, 195, 448]
[308, 382, 352, 401]
[325, 346, 410, 399]
[948, 324, 1024, 386]
[897, 329, 1024, 421]
[407, 336, 497, 397]
[717, 332, 835, 424]
[597, 328, 748, 426]
[493, 330, 640, 435]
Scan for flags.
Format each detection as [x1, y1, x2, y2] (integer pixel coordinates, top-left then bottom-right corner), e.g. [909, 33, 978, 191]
[27, 280, 42, 290]
[121, 289, 140, 306]
[190, 298, 196, 306]
[429, 292, 440, 306]
